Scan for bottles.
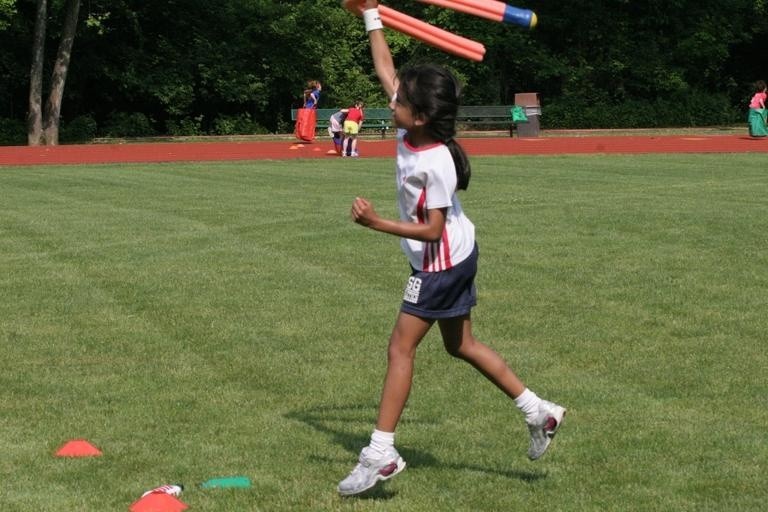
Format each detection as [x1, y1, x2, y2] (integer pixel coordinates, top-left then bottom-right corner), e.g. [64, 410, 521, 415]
[142, 484, 184, 499]
[200, 477, 250, 489]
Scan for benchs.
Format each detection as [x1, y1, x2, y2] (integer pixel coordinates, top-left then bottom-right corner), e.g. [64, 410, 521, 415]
[291, 107, 400, 140]
[456, 105, 516, 137]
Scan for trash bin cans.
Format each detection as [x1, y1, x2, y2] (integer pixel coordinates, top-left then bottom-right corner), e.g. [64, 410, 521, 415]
[514, 93, 541, 137]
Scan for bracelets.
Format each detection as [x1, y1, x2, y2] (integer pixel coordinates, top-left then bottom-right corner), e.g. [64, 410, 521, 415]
[364, 8, 384, 32]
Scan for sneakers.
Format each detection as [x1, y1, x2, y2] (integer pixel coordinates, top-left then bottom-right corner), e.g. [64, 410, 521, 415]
[336, 447, 406, 496]
[524, 400, 566, 462]
[327, 127, 335, 138]
[341, 151, 359, 157]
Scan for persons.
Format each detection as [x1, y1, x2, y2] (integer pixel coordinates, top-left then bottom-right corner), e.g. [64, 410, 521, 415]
[303, 81, 322, 109]
[336, 0, 566, 496]
[749, 80, 767, 109]
[328, 98, 364, 158]
